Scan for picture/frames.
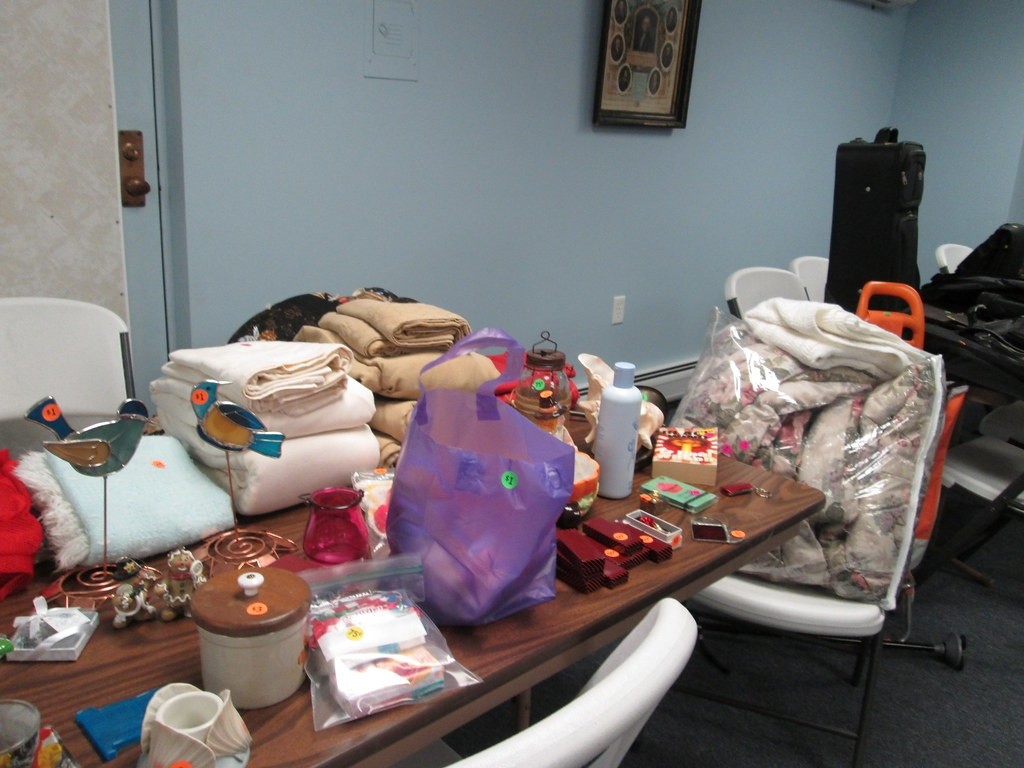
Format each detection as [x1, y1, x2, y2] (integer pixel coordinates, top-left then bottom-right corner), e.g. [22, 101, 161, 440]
[591, 0, 701, 129]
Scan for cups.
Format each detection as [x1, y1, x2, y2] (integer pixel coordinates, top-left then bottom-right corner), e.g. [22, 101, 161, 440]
[299, 488, 369, 565]
[635, 385, 667, 462]
[0, 698, 40, 768]
[136, 682, 252, 768]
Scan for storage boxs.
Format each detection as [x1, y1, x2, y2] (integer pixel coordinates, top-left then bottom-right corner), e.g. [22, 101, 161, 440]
[556, 517, 672, 596]
[6, 610, 99, 661]
[622, 509, 682, 550]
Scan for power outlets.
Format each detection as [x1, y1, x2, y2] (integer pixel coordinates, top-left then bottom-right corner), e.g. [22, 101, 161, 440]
[611, 295, 626, 324]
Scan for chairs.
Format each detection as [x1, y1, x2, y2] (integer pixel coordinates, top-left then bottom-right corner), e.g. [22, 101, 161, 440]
[722, 244, 1024, 586]
[0, 297, 137, 431]
[665, 297, 947, 767]
[394, 596, 698, 767]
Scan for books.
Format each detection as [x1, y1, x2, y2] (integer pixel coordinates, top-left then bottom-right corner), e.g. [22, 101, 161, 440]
[652, 427, 718, 485]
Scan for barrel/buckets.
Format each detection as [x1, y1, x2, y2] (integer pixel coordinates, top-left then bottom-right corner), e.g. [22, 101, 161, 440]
[191, 567, 312, 708]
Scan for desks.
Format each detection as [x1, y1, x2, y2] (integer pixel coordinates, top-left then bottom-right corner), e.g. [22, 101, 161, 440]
[0, 402, 825, 768]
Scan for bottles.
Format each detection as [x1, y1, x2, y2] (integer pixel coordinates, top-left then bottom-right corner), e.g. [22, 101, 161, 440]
[509, 331, 571, 443]
[595, 361, 642, 499]
